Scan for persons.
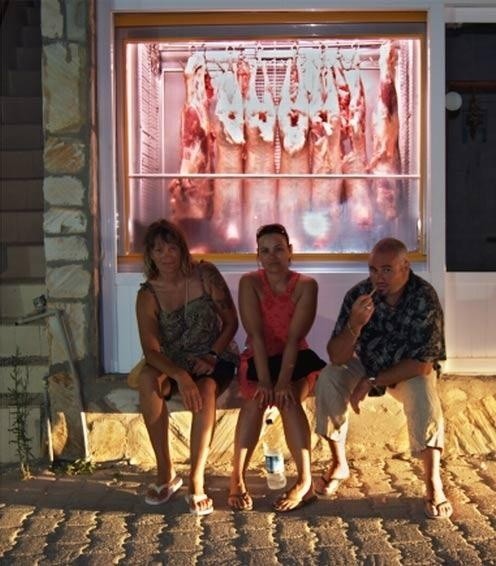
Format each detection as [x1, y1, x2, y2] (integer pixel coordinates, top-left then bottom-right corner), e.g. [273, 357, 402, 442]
[227, 222, 328, 514]
[313, 237, 454, 521]
[133, 219, 241, 517]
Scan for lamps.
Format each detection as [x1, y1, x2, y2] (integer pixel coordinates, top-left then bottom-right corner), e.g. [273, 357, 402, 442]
[446, 92, 463, 112]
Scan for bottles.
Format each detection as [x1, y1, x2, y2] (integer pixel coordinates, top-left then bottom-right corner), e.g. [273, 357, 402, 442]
[262, 419, 287, 490]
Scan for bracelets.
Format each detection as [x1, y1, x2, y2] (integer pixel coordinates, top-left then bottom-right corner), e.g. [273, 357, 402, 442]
[346, 319, 362, 339]
[207, 350, 222, 361]
[368, 376, 378, 390]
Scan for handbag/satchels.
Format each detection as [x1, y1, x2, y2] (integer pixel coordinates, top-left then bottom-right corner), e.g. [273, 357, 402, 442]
[127, 358, 147, 389]
[246, 348, 327, 380]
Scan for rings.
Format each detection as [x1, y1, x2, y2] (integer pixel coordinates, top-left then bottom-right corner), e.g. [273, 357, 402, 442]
[365, 305, 372, 312]
[285, 396, 290, 400]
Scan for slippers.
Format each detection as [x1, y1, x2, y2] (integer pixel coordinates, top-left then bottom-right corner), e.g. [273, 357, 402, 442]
[313, 472, 354, 499]
[270, 491, 318, 513]
[184, 494, 214, 516]
[422, 490, 453, 518]
[228, 490, 254, 511]
[144, 477, 183, 506]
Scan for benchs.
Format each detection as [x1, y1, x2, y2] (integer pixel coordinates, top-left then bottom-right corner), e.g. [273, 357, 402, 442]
[88, 372, 496, 480]
